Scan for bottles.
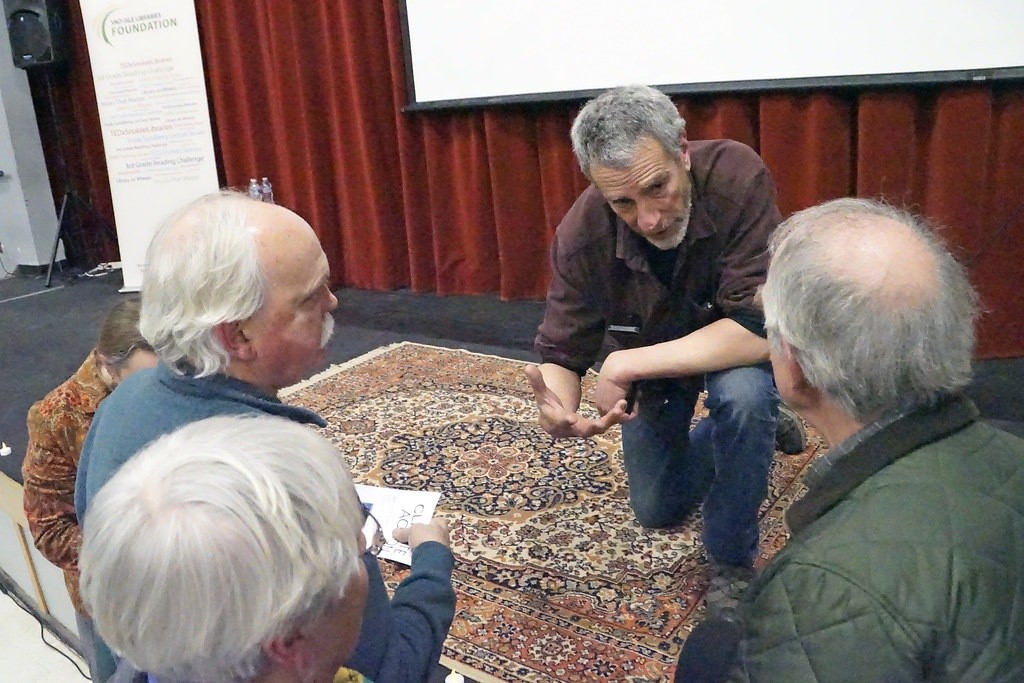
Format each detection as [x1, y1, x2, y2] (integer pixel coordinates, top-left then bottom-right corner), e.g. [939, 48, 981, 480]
[445, 672, 464, 683]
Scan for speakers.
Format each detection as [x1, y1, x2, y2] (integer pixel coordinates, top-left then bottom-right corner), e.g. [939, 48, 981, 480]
[3, 0, 73, 70]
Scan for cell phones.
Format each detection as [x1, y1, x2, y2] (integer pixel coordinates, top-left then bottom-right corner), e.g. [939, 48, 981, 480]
[607, 325, 641, 337]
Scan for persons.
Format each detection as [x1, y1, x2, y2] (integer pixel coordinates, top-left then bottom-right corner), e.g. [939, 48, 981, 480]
[78, 410, 378, 683]
[674, 198, 1023, 683]
[22, 301, 164, 683]
[526, 83, 807, 623]
[72, 190, 457, 683]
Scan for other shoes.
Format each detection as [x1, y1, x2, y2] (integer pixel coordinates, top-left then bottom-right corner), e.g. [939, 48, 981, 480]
[705, 551, 757, 622]
[775, 400, 806, 454]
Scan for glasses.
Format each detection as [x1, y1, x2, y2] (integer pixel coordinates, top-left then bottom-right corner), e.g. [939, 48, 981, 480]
[356, 505, 385, 558]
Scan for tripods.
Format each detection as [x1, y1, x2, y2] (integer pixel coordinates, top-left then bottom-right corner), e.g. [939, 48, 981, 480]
[42, 71, 118, 288]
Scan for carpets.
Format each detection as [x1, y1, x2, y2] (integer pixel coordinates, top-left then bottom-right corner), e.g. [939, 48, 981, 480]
[277, 341, 831, 683]
